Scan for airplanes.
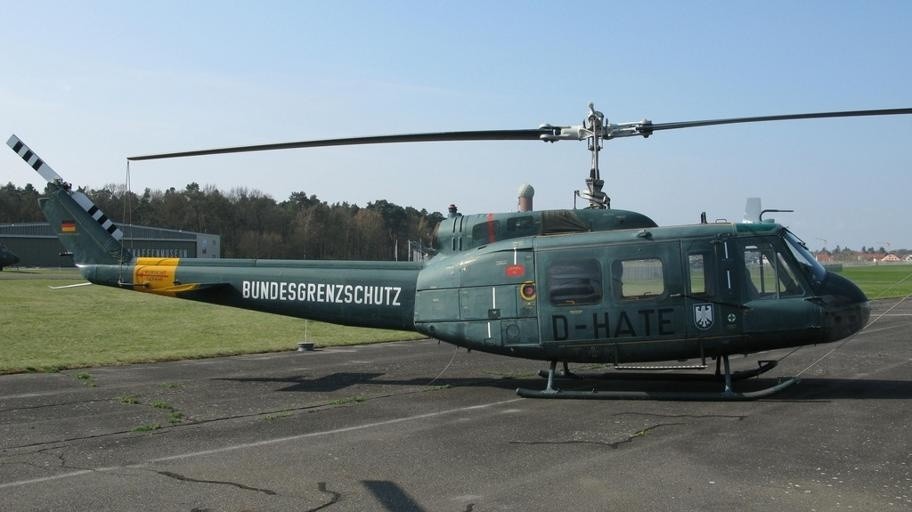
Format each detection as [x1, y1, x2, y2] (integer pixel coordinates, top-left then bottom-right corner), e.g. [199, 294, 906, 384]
[0, 243, 20, 271]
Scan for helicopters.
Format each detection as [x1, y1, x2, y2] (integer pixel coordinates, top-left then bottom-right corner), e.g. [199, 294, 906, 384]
[6, 101, 912, 400]
